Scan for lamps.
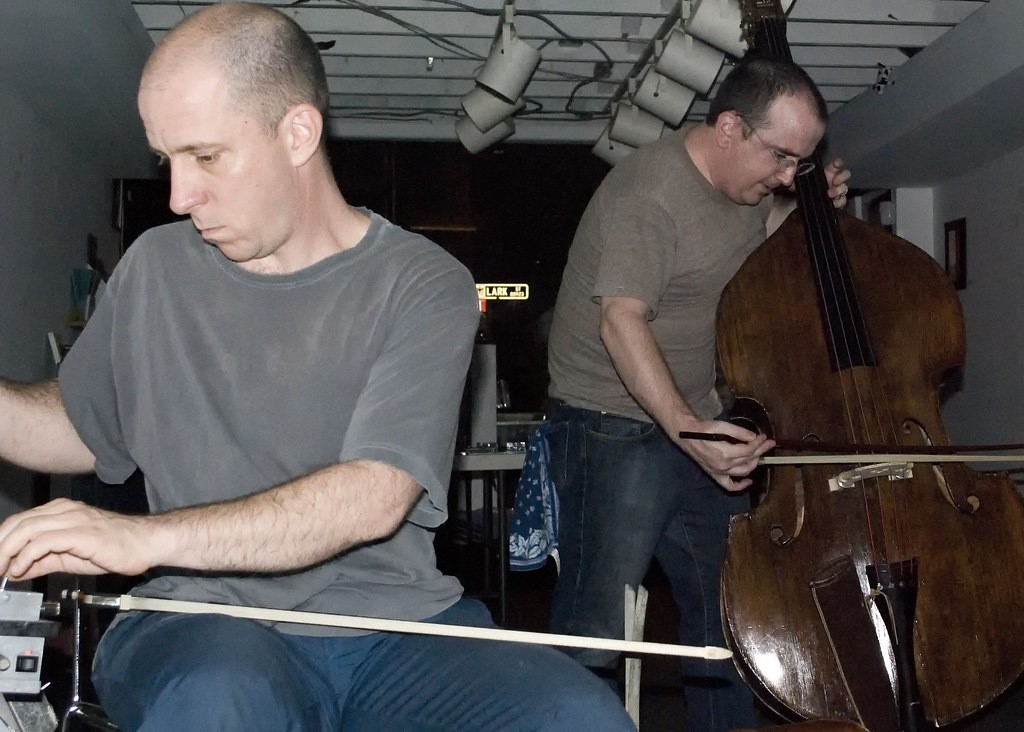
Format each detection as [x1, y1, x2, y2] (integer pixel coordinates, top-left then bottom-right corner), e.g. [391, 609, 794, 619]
[460, 85, 525, 133]
[627, 65, 696, 128]
[590, 122, 637, 165]
[654, 29, 725, 95]
[474, 6, 542, 105]
[680, 0, 751, 58]
[606, 102, 664, 148]
[456, 114, 516, 155]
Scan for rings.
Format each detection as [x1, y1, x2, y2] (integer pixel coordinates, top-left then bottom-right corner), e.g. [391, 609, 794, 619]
[841, 189, 848, 195]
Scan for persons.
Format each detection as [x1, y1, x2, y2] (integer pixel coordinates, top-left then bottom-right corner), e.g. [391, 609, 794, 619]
[547, 53, 851, 732]
[1, 0, 640, 731]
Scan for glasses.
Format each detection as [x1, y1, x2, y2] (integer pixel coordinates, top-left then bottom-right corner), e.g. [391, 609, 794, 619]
[738, 114, 815, 176]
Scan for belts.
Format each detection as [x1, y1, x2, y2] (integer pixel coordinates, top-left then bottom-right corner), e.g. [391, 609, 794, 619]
[549, 404, 602, 422]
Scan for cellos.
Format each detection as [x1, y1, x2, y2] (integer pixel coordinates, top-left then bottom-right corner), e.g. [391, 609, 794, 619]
[719, 0, 1024, 732]
